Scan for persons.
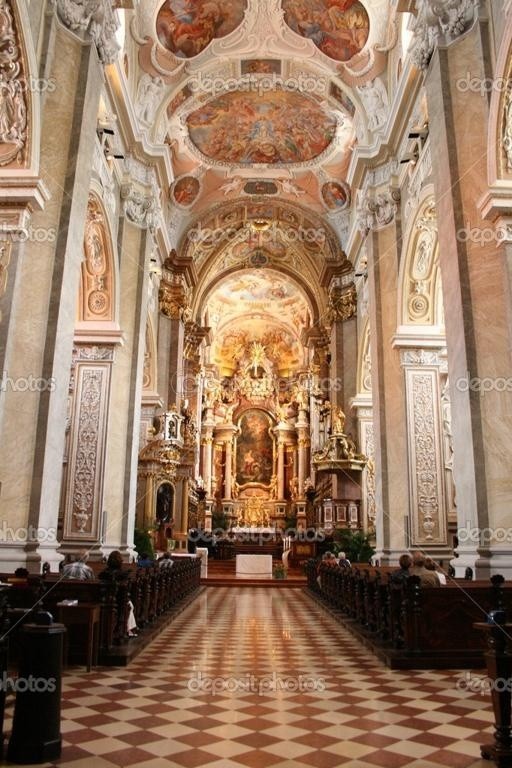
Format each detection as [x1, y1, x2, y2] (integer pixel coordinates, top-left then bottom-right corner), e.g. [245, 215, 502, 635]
[98, 550, 139, 638]
[138, 552, 153, 566]
[387, 555, 413, 581]
[160, 0, 370, 203]
[315, 551, 353, 591]
[400, 552, 440, 586]
[159, 553, 174, 568]
[60, 549, 96, 580]
[426, 558, 447, 584]
[239, 411, 271, 474]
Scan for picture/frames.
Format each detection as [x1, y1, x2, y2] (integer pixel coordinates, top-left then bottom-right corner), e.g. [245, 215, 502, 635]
[315, 504, 358, 523]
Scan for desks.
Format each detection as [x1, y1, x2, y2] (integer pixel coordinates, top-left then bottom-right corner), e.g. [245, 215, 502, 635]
[56, 600, 102, 673]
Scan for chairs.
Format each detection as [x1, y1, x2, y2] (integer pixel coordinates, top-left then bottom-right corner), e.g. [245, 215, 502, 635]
[1, 556, 201, 651]
[308, 560, 510, 657]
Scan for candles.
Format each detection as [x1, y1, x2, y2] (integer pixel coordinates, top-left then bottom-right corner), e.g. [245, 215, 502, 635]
[283, 536, 291, 551]
